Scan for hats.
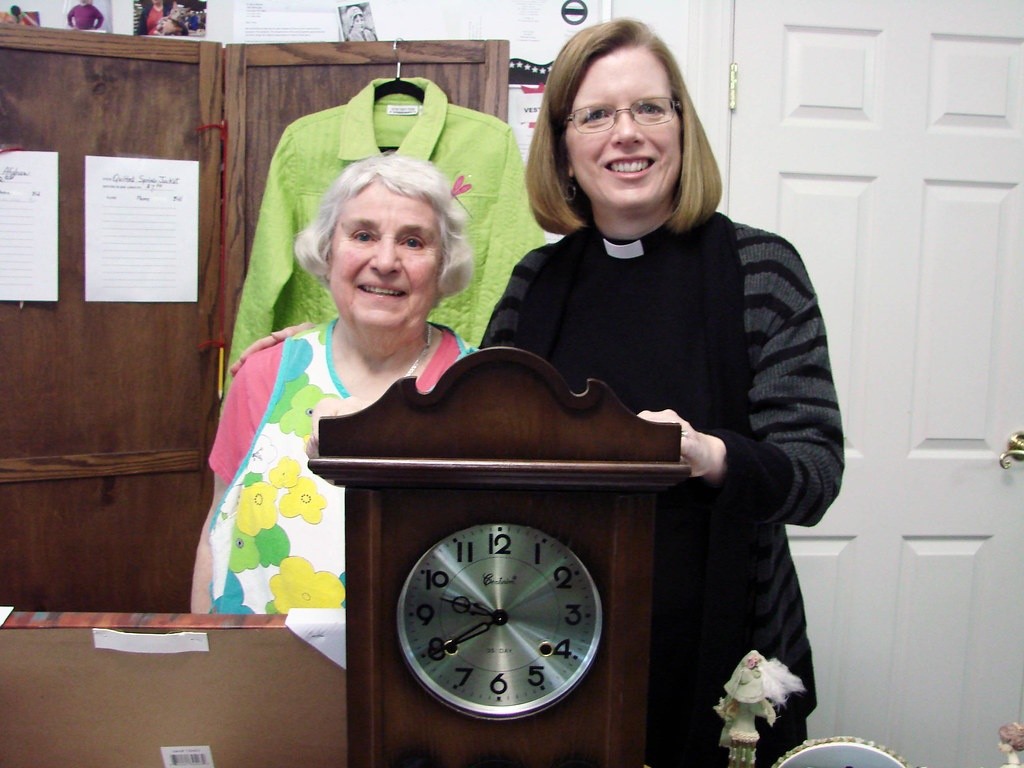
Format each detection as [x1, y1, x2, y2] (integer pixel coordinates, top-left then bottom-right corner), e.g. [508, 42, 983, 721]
[343, 5, 364, 27]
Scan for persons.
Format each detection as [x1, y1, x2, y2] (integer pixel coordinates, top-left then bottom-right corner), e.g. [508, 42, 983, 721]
[343, 6, 376, 41]
[188, 154, 480, 620]
[996, 721, 1024, 768]
[137, 0, 198, 38]
[66, 0, 104, 29]
[474, 18, 848, 766]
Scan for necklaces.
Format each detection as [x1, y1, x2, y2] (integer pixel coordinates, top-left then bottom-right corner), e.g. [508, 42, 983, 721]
[403, 323, 434, 377]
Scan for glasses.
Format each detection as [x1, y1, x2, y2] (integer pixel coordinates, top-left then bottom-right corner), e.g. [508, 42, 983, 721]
[564, 96, 683, 136]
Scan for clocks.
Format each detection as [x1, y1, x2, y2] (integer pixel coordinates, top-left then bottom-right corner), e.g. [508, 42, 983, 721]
[307, 342, 695, 768]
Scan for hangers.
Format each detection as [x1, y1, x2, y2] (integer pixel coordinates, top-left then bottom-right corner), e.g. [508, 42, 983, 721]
[373, 37, 426, 153]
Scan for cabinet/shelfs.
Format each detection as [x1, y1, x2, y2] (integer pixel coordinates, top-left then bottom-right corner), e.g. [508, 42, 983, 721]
[0, 21, 513, 613]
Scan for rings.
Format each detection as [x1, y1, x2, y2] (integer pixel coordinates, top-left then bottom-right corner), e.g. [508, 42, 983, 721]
[681, 429, 688, 437]
